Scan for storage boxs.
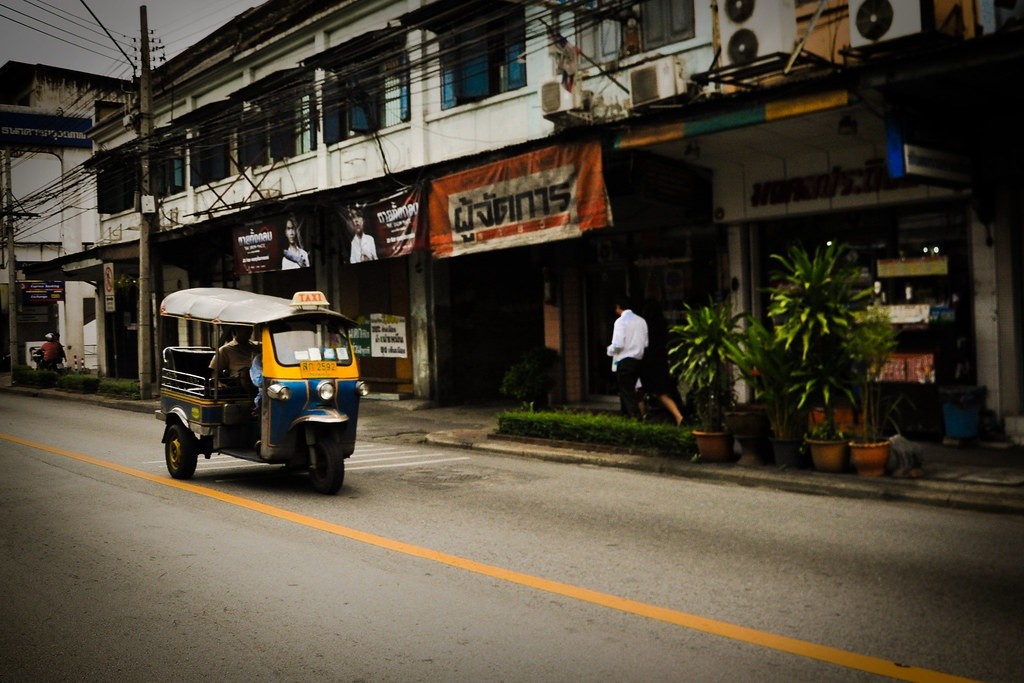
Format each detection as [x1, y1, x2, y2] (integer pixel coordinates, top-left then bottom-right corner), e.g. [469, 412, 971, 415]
[871, 256, 948, 381]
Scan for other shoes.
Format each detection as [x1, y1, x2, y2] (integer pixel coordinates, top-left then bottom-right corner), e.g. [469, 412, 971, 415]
[256, 441, 261, 456]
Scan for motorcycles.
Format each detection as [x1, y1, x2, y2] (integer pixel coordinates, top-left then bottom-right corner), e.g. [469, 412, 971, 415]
[159, 286, 372, 495]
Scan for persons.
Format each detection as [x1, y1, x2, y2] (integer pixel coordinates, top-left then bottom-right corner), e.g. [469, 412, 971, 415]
[281, 213, 311, 270]
[606, 297, 687, 427]
[37, 332, 67, 369]
[345, 206, 378, 264]
[208, 320, 291, 453]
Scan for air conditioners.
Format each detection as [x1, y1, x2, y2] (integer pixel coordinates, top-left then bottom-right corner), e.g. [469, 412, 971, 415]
[629, 54, 688, 108]
[537, 75, 585, 121]
[717, 0, 935, 75]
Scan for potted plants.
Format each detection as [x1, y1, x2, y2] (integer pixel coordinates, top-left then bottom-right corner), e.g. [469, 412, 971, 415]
[667, 240, 920, 480]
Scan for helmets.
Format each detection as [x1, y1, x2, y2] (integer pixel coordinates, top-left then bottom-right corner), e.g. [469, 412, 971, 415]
[45, 333, 54, 340]
